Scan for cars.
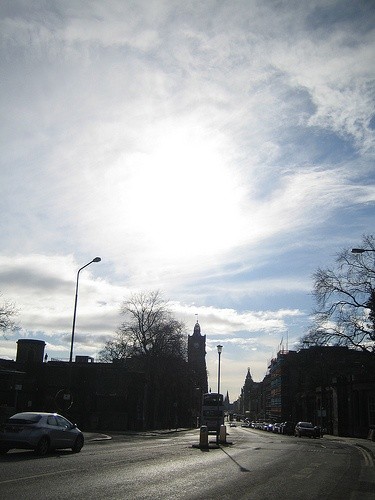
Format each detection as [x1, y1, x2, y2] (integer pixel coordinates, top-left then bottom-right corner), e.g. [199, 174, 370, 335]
[230, 417, 319, 439]
[0, 412, 84, 458]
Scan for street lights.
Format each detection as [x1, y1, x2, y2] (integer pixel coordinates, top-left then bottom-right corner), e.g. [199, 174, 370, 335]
[69, 256, 102, 362]
[216, 344, 223, 394]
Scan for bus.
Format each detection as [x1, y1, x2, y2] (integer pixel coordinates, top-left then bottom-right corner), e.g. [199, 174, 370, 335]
[203, 391, 224, 435]
